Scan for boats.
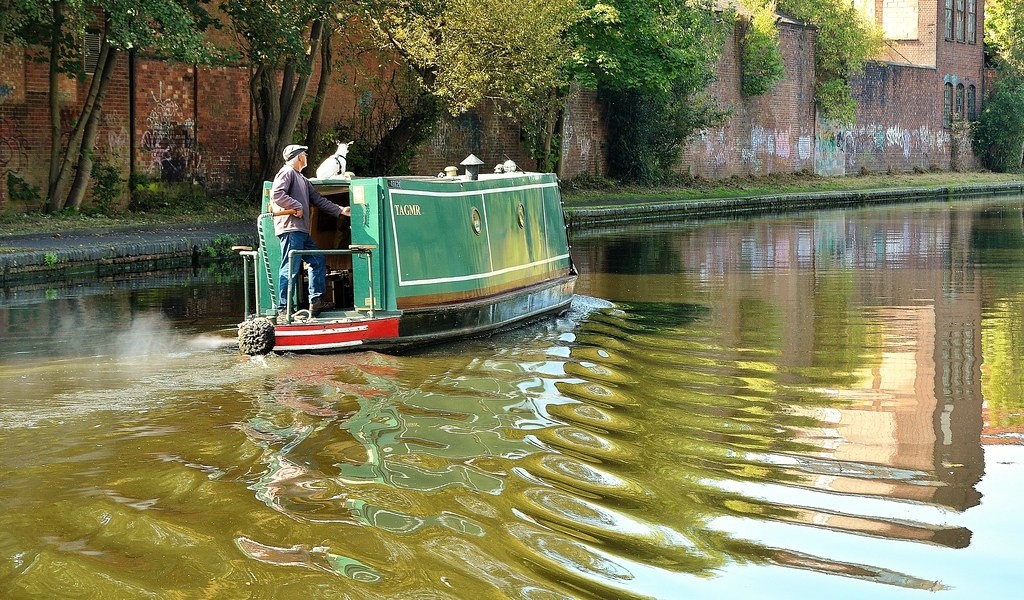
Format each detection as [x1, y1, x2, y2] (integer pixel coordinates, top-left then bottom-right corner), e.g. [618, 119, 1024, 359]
[233, 153, 580, 353]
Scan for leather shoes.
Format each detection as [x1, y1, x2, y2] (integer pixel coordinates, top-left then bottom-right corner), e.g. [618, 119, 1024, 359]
[309, 299, 335, 316]
[281, 307, 305, 319]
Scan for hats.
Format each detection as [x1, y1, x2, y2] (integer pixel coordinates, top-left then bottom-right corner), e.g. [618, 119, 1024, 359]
[283, 145, 308, 161]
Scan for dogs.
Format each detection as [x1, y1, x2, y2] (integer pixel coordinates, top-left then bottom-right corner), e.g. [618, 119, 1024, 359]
[316, 138, 354, 180]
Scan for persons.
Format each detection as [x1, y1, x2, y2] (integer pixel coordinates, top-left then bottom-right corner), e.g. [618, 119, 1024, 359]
[270, 144, 351, 318]
